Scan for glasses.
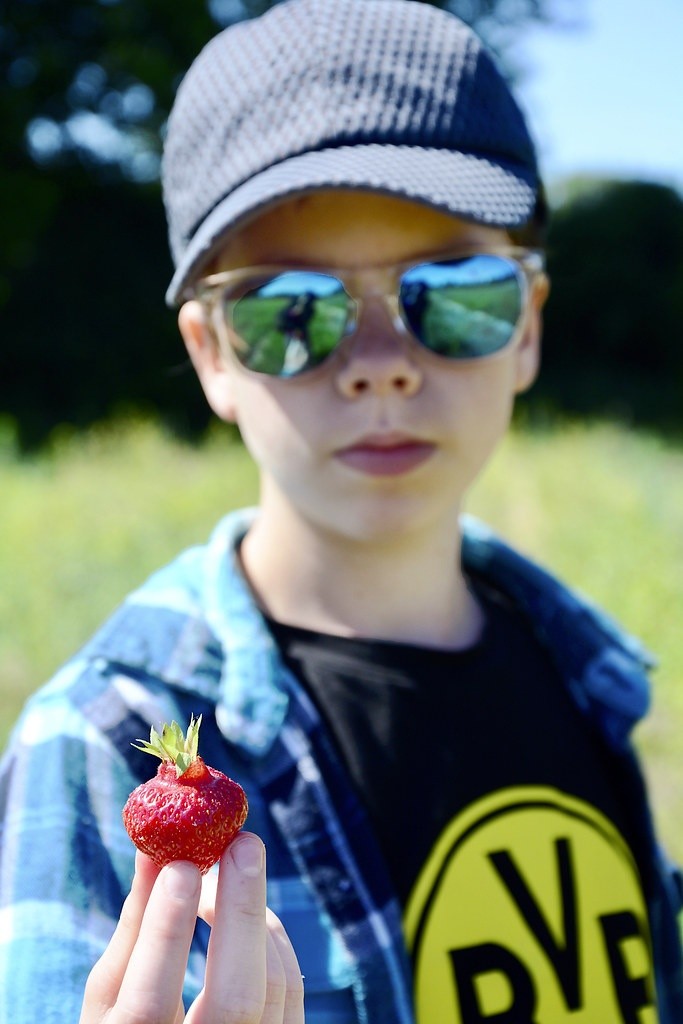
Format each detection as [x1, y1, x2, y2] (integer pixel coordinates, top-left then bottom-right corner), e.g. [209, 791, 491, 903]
[187, 248, 543, 379]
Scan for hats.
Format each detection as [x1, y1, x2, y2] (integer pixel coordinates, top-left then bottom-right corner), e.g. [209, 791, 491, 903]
[159, 0, 549, 307]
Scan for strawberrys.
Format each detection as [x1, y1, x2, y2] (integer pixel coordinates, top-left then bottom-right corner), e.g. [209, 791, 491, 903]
[120, 714, 248, 875]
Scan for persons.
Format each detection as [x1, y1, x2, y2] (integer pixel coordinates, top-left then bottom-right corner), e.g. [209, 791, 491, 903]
[0, 0, 683, 1022]
